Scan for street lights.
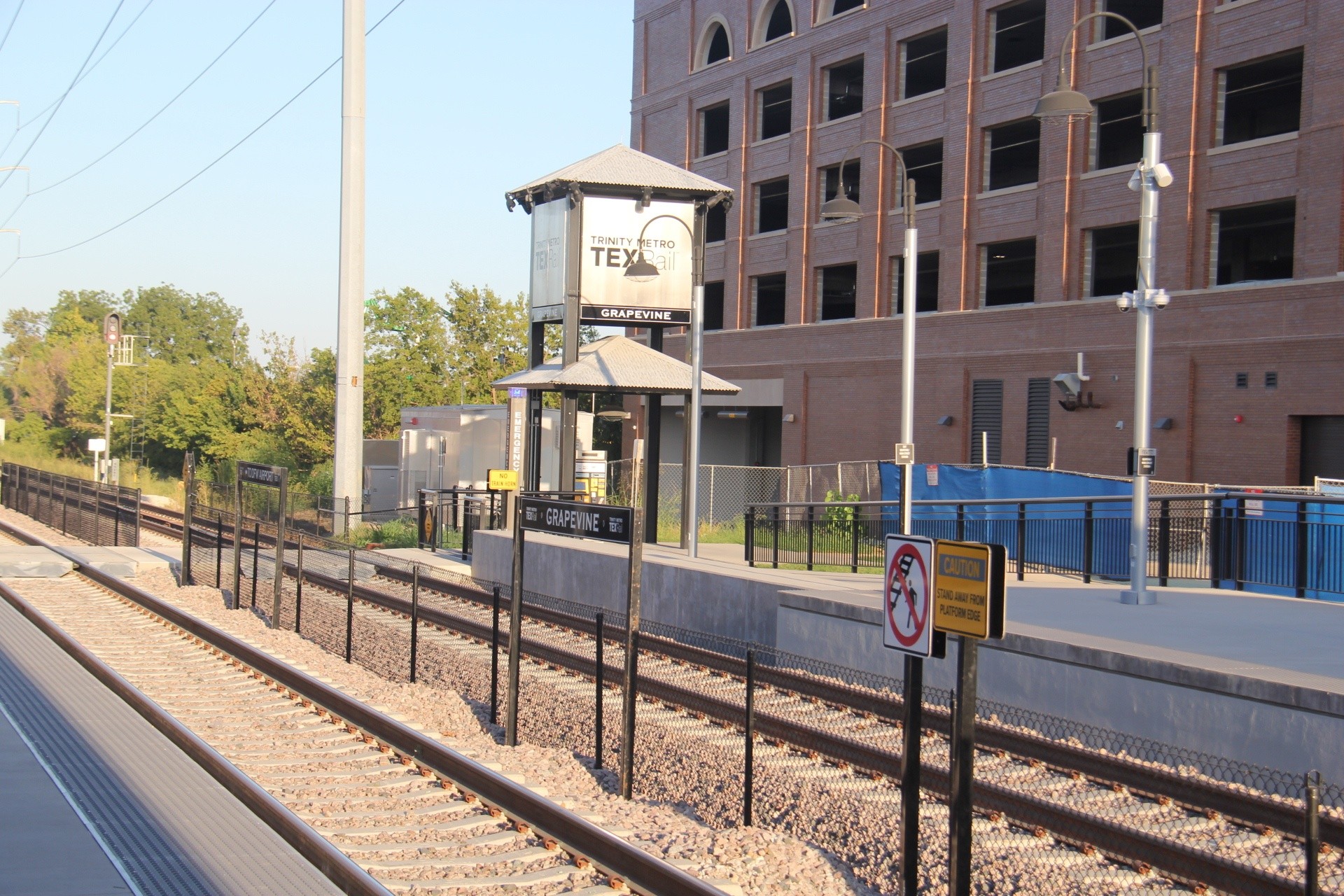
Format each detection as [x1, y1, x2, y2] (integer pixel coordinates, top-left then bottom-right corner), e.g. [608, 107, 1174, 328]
[1030, 10, 1173, 605]
[623, 214, 706, 559]
[817, 140, 919, 536]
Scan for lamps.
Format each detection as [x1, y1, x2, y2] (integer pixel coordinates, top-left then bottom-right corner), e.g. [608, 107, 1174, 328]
[721, 192, 734, 214]
[505, 192, 518, 213]
[524, 186, 539, 209]
[569, 181, 585, 203]
[639, 186, 654, 207]
[543, 182, 561, 203]
[705, 192, 724, 209]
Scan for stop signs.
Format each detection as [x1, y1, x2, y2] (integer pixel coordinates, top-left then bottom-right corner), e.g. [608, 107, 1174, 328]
[489, 469, 518, 490]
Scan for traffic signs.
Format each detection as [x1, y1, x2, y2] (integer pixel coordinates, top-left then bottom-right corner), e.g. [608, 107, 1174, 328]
[236, 460, 282, 489]
[519, 497, 633, 546]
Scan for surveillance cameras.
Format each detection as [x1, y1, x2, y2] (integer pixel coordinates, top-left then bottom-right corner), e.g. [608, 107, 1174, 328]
[1144, 288, 1170, 310]
[1116, 290, 1138, 313]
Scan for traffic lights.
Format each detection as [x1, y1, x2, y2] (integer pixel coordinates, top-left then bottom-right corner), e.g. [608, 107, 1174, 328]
[103, 313, 121, 345]
